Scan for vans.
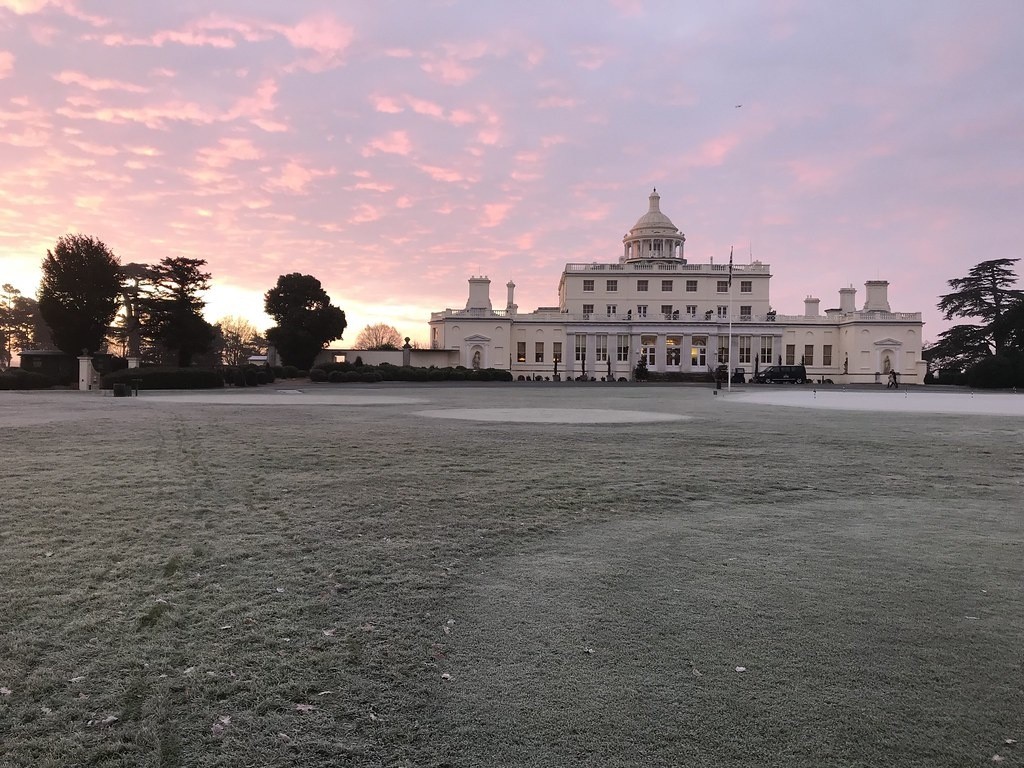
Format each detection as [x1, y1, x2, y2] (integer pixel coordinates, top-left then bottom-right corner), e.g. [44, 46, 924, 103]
[756, 365, 807, 384]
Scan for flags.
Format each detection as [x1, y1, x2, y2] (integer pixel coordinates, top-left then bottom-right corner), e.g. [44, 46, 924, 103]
[728, 251, 733, 289]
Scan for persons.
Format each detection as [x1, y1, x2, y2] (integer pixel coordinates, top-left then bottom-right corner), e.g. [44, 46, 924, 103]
[890, 369, 899, 388]
[886, 370, 897, 389]
